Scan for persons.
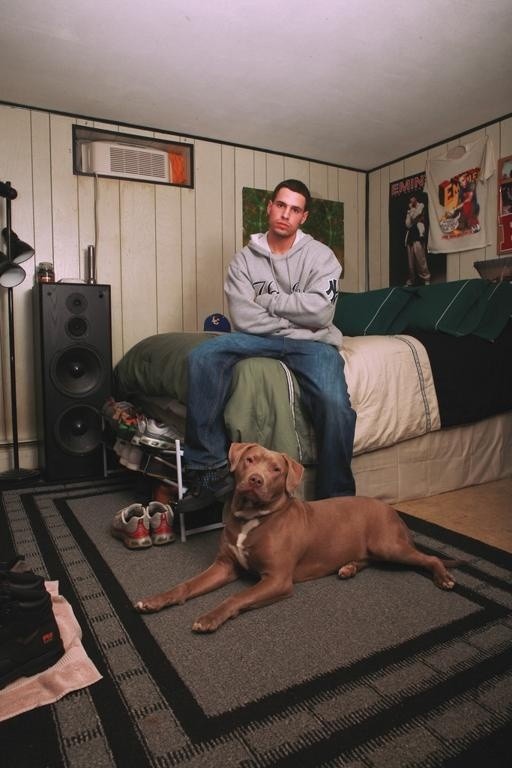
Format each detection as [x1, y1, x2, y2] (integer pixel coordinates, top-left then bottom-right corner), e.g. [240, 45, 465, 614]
[175, 179, 356, 513]
[449, 175, 480, 234]
[404, 196, 432, 286]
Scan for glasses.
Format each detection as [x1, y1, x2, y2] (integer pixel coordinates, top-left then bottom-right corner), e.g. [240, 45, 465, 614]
[113, 501, 177, 549]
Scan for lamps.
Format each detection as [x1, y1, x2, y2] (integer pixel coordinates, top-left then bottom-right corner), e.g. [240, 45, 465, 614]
[0, 180, 46, 486]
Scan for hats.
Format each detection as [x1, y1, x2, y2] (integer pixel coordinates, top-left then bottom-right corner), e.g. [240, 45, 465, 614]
[204, 313, 230, 331]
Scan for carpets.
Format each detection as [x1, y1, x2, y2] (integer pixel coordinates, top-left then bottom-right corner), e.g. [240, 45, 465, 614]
[0, 468, 512, 768]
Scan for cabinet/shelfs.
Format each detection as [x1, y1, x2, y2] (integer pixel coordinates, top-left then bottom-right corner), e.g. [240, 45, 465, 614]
[102, 416, 225, 544]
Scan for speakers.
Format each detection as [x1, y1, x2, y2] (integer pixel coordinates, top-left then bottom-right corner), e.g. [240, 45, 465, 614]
[32, 281, 115, 482]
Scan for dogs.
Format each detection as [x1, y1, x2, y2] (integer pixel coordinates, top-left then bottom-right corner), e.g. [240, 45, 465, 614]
[131, 441, 459, 636]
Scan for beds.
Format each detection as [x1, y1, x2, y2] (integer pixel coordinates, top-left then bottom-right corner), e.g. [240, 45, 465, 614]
[119, 278, 511, 509]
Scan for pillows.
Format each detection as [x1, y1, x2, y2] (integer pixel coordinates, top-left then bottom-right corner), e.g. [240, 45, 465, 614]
[333, 279, 511, 343]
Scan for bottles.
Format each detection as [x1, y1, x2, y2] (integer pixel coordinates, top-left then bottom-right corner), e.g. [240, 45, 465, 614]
[37, 261, 56, 285]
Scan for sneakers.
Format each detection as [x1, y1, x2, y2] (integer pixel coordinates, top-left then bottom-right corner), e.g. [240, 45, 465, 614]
[100, 398, 184, 480]
[176, 470, 234, 513]
[0, 555, 65, 690]
